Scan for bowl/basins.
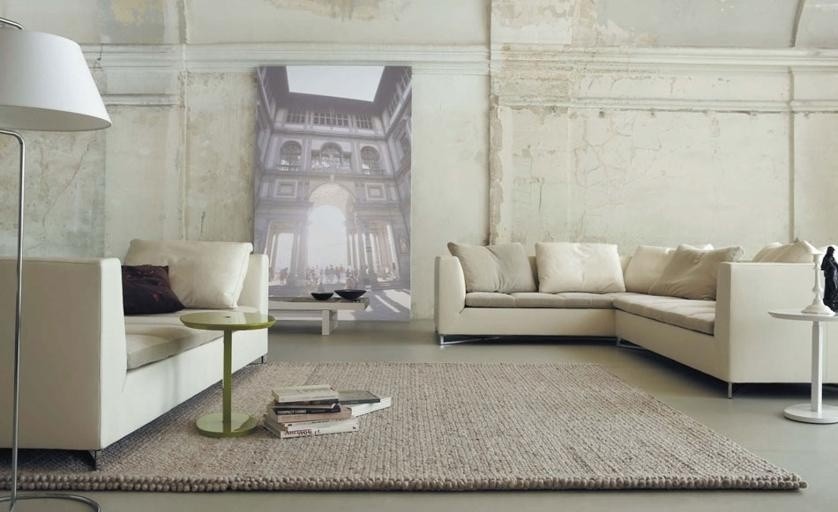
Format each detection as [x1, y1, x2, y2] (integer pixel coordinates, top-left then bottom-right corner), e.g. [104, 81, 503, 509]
[311, 292, 333, 301]
[333, 289, 367, 300]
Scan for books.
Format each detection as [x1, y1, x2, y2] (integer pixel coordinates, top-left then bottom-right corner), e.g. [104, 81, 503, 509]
[260, 383, 393, 440]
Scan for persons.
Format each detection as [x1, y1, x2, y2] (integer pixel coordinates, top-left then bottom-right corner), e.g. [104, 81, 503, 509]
[818, 245, 838, 312]
[268, 260, 395, 291]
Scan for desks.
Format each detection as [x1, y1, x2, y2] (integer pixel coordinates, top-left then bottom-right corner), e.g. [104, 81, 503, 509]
[268, 298, 370, 335]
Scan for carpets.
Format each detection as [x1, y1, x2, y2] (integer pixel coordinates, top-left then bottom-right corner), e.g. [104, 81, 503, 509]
[1, 361, 806, 492]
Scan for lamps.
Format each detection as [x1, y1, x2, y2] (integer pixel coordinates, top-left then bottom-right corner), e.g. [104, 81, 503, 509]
[0, 17, 114, 512]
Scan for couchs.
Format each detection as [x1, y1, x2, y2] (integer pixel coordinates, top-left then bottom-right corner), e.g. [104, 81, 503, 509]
[435, 255, 837, 400]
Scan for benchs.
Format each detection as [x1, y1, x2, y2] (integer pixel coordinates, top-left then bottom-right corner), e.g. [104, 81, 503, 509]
[0, 254, 270, 471]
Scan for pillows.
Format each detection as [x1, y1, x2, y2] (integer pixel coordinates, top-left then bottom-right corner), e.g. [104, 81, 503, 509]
[122, 239, 254, 315]
